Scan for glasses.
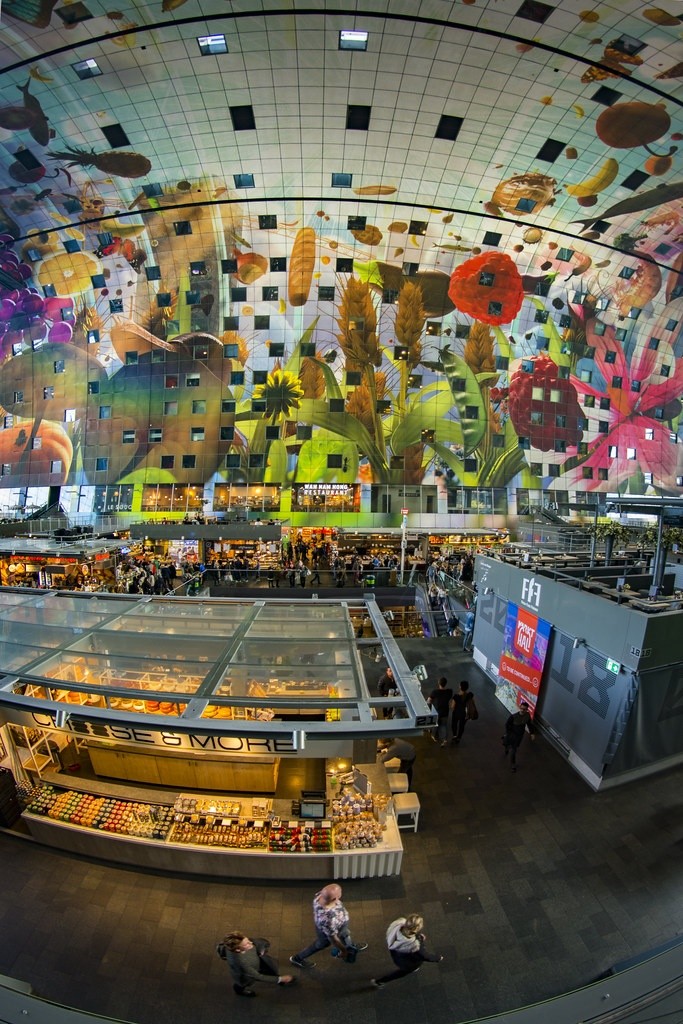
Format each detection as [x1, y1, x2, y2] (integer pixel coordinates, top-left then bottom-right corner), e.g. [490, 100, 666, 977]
[520, 708, 528, 710]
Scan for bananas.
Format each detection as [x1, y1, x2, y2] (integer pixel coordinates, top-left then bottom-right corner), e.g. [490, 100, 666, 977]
[101, 211, 145, 237]
[562, 158, 619, 197]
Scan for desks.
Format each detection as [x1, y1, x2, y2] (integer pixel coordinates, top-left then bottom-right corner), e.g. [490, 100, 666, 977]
[21, 803, 334, 880]
[531, 556, 555, 566]
[554, 556, 579, 567]
[582, 580, 609, 589]
[602, 589, 641, 604]
[333, 807, 404, 879]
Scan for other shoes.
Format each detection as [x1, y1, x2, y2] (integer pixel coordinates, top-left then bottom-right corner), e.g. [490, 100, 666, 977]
[235, 990, 255, 997]
[353, 942, 368, 950]
[371, 979, 384, 989]
[289, 953, 316, 970]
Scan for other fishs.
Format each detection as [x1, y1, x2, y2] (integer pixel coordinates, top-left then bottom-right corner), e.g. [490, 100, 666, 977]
[17, 77, 52, 147]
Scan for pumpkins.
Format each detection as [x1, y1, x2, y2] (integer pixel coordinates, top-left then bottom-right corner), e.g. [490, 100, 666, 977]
[81, 350, 234, 482]
[0, 420, 73, 485]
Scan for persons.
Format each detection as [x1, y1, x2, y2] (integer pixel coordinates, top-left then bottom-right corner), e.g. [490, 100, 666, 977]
[425, 677, 453, 747]
[223, 931, 293, 997]
[288, 884, 368, 968]
[449, 681, 474, 743]
[503, 700, 536, 773]
[371, 913, 444, 989]
[376, 666, 399, 720]
[0, 511, 531, 637]
[377, 737, 416, 786]
[462, 606, 476, 652]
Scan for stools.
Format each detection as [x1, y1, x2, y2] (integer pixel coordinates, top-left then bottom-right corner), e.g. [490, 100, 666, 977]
[393, 792, 420, 833]
[387, 773, 408, 793]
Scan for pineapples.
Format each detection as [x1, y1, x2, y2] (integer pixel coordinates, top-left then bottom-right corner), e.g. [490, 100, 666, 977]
[45, 147, 151, 178]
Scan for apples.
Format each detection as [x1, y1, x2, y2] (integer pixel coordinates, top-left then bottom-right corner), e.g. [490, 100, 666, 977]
[232, 253, 267, 285]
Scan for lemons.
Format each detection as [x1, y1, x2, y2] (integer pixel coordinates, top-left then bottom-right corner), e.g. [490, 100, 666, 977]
[33, 250, 103, 300]
[21, 214, 85, 266]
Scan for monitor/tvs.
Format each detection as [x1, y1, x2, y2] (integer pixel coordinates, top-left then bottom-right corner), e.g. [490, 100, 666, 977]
[300, 791, 326, 798]
[298, 802, 326, 819]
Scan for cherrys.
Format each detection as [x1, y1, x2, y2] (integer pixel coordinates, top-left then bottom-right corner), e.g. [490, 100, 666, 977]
[0, 234, 75, 362]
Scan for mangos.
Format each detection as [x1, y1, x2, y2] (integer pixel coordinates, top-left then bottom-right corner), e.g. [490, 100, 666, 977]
[0, 342, 108, 421]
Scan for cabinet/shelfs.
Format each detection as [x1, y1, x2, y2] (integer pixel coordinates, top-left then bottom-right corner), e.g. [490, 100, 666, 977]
[100, 670, 233, 719]
[87, 740, 277, 793]
[7, 657, 106, 778]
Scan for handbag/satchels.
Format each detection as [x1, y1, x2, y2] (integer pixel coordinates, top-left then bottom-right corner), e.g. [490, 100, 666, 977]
[466, 698, 479, 720]
